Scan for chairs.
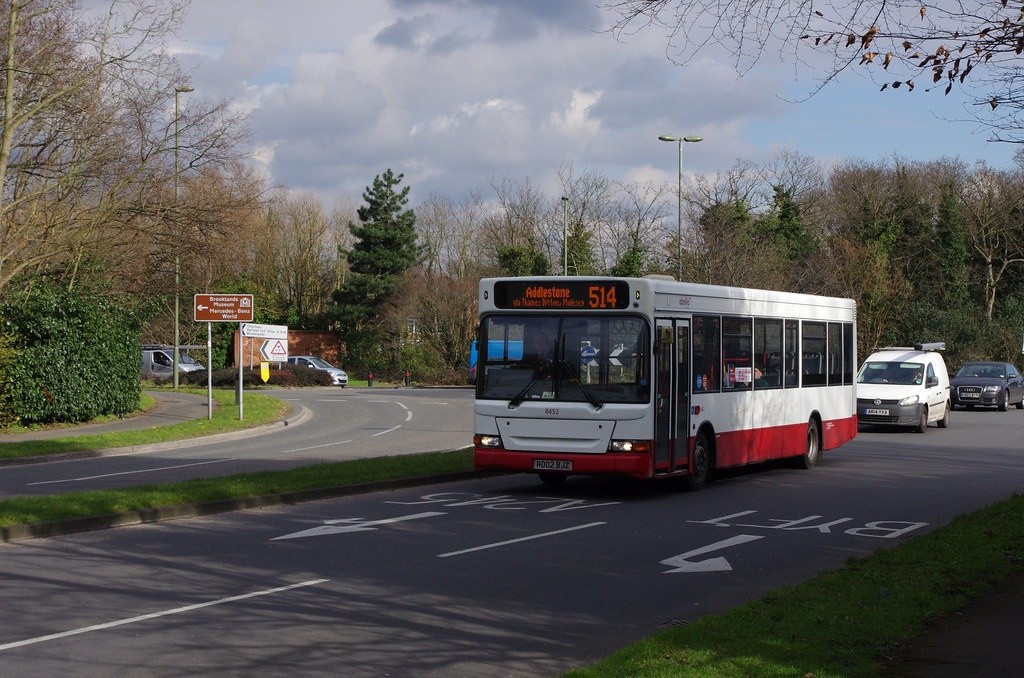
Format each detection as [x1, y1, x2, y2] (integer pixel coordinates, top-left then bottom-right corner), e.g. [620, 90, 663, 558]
[991, 368, 1002, 378]
[733, 350, 835, 386]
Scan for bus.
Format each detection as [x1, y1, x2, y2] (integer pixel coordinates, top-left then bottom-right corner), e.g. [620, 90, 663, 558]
[468, 339, 525, 383]
[471, 274, 859, 499]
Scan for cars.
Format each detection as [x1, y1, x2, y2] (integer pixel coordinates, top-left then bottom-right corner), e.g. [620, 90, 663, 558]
[270, 355, 348, 388]
[950, 361, 1024, 412]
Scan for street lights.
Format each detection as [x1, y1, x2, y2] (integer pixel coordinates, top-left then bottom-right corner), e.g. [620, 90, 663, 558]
[659, 133, 704, 284]
[558, 195, 572, 276]
[173, 86, 197, 391]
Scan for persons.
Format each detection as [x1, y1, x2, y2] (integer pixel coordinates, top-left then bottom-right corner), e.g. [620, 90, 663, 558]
[743, 363, 769, 388]
[517, 331, 559, 379]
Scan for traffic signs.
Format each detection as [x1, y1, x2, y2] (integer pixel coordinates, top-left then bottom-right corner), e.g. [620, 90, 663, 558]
[192, 293, 254, 323]
[242, 323, 288, 340]
[259, 339, 289, 362]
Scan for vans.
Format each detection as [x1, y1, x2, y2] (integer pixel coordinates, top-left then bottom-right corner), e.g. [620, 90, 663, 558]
[139, 349, 206, 381]
[857, 341, 952, 432]
